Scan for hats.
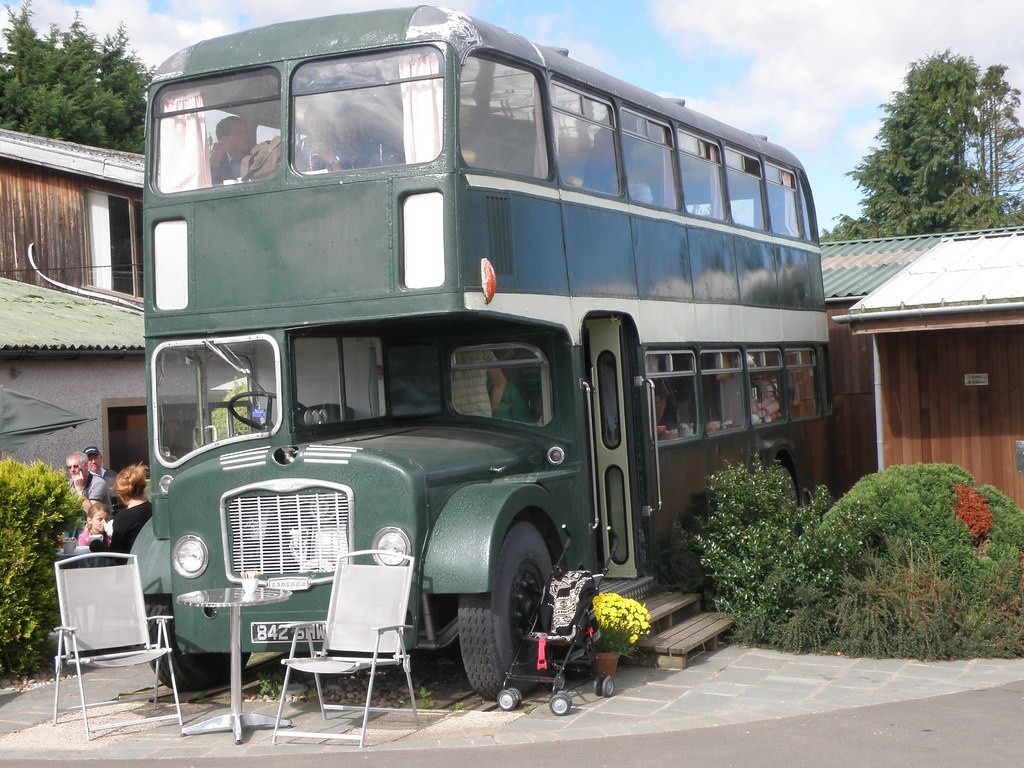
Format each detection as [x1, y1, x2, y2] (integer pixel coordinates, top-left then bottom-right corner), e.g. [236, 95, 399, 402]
[84, 446, 99, 455]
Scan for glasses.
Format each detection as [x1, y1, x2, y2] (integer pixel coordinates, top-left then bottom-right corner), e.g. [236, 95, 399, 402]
[67, 465, 79, 470]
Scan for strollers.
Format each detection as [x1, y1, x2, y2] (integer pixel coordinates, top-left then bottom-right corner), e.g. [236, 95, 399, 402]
[498, 525, 620, 718]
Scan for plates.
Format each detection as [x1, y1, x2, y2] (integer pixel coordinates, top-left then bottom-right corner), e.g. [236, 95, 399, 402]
[56, 551, 81, 557]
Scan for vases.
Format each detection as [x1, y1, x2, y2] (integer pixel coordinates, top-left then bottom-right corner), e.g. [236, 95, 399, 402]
[593, 653, 621, 678]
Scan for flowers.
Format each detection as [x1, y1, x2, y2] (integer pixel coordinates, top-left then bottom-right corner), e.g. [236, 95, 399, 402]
[591, 593, 651, 659]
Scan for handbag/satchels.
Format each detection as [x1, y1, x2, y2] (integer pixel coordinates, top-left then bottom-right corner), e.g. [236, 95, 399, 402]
[241, 135, 282, 181]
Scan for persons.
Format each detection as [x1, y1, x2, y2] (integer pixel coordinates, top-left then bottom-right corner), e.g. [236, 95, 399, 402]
[453, 350, 506, 417]
[609, 163, 653, 205]
[65, 446, 152, 565]
[296, 106, 404, 173]
[210, 116, 249, 183]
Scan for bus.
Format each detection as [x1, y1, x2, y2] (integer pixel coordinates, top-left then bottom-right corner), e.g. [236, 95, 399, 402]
[121, 2, 841, 706]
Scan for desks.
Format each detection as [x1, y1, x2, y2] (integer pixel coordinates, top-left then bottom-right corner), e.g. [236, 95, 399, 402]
[176, 587, 293, 745]
[56, 545, 114, 569]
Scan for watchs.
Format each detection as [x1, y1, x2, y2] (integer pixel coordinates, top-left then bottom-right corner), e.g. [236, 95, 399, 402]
[78, 490, 87, 496]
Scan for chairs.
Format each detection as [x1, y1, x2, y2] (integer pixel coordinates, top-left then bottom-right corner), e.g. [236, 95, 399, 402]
[270, 550, 418, 748]
[51, 551, 182, 743]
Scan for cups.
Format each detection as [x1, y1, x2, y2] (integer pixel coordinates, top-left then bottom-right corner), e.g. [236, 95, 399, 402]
[678, 422, 695, 437]
[63, 541, 77, 554]
[705, 421, 722, 432]
[89, 534, 104, 545]
[240, 578, 259, 594]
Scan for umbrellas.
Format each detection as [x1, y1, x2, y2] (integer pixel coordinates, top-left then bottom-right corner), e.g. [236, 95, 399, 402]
[0, 385, 98, 461]
